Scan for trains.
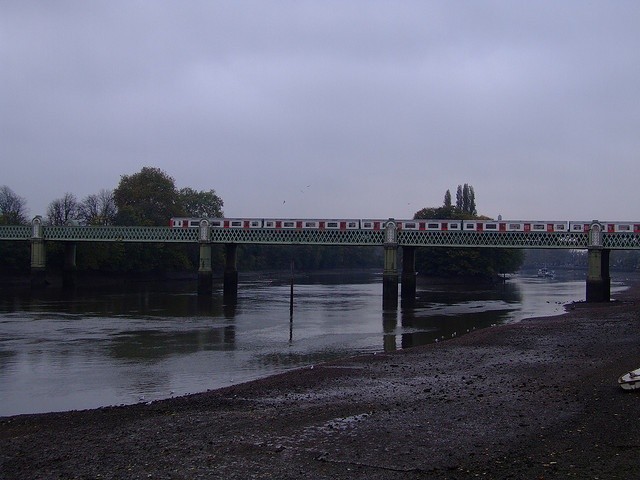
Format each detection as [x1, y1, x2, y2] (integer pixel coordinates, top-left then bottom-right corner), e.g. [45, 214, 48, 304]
[170, 217, 640, 243]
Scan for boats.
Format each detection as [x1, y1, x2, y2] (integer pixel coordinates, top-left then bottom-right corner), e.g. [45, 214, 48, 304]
[538, 263, 557, 279]
